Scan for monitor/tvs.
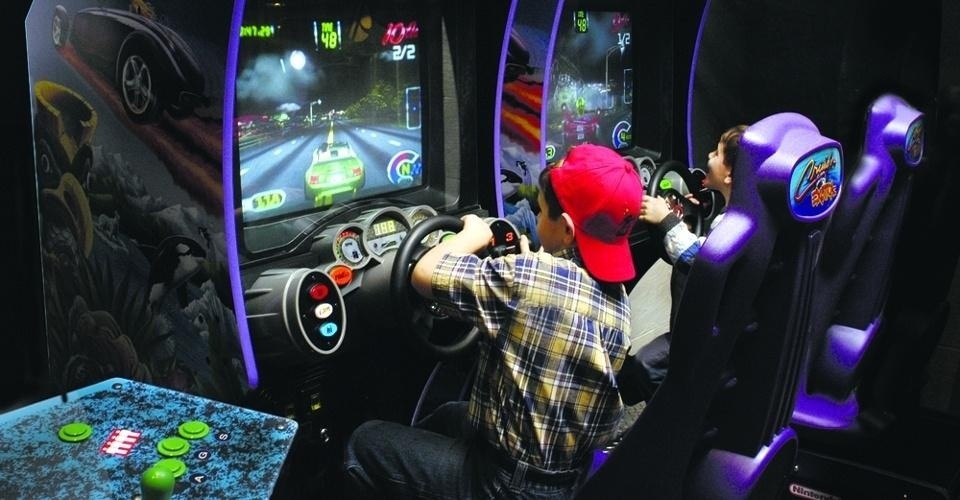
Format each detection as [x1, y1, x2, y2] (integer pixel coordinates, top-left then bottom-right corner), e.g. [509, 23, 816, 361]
[545, 9, 635, 167]
[234, 0, 427, 225]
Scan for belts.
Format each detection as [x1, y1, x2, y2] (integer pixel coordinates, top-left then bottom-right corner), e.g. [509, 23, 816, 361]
[501, 464, 576, 486]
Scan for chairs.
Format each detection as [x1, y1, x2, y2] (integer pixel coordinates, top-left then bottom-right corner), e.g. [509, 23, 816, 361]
[784, 92, 928, 431]
[579, 111, 835, 494]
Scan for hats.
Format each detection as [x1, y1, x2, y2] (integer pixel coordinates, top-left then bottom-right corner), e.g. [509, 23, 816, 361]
[549, 144, 643, 283]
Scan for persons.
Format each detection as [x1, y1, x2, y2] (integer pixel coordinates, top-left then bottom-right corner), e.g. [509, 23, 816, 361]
[634, 125, 749, 379]
[341, 144, 643, 499]
